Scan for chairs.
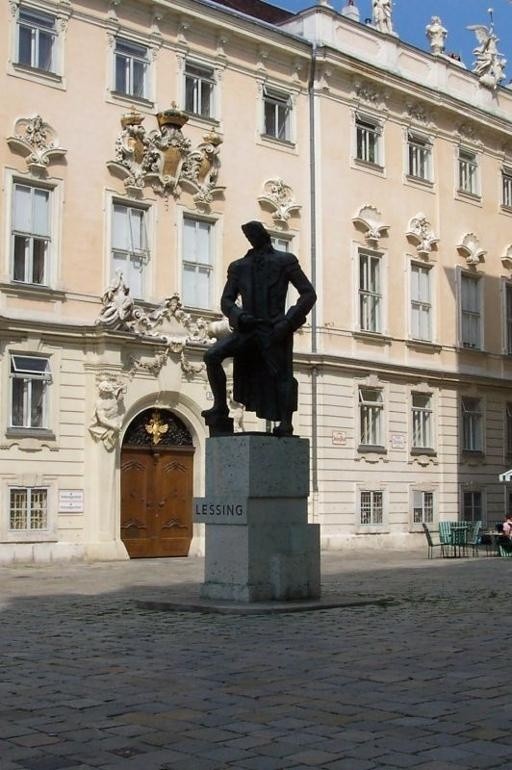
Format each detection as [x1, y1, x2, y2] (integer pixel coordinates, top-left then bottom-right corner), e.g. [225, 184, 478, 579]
[422, 520, 511, 560]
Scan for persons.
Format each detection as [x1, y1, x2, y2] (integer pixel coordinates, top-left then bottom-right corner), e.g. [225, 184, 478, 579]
[201, 220, 316, 434]
[426, 16, 447, 52]
[493, 513, 512, 557]
